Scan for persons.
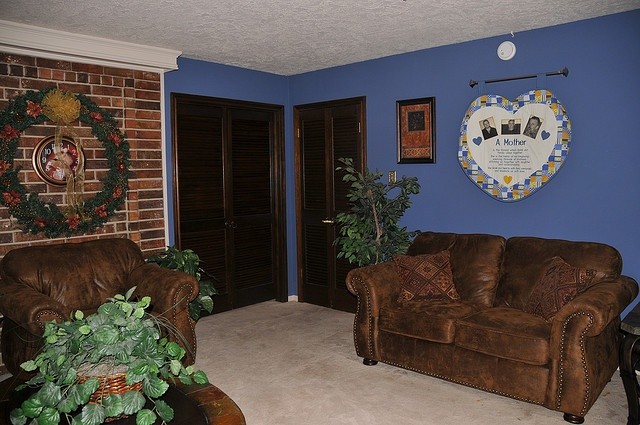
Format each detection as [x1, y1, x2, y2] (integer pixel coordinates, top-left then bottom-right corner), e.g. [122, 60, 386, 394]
[481, 120, 498, 140]
[501, 120, 520, 134]
[523, 116, 541, 139]
